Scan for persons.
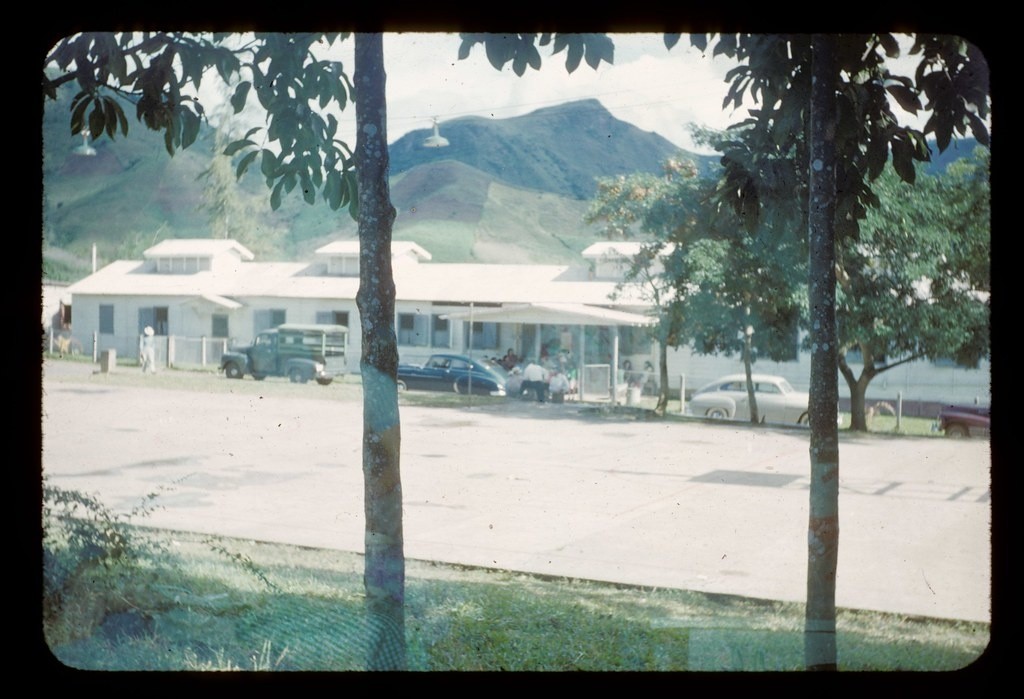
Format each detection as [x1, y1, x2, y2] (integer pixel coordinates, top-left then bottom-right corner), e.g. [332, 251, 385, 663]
[481, 337, 655, 403]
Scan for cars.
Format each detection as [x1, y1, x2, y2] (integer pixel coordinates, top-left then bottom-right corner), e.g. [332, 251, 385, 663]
[397, 353, 506, 395]
[690, 374, 841, 428]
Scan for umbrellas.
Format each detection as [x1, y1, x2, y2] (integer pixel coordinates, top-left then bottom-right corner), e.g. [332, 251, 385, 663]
[438, 303, 659, 328]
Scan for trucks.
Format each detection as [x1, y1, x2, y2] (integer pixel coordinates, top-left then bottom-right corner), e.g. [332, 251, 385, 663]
[217, 323, 348, 386]
